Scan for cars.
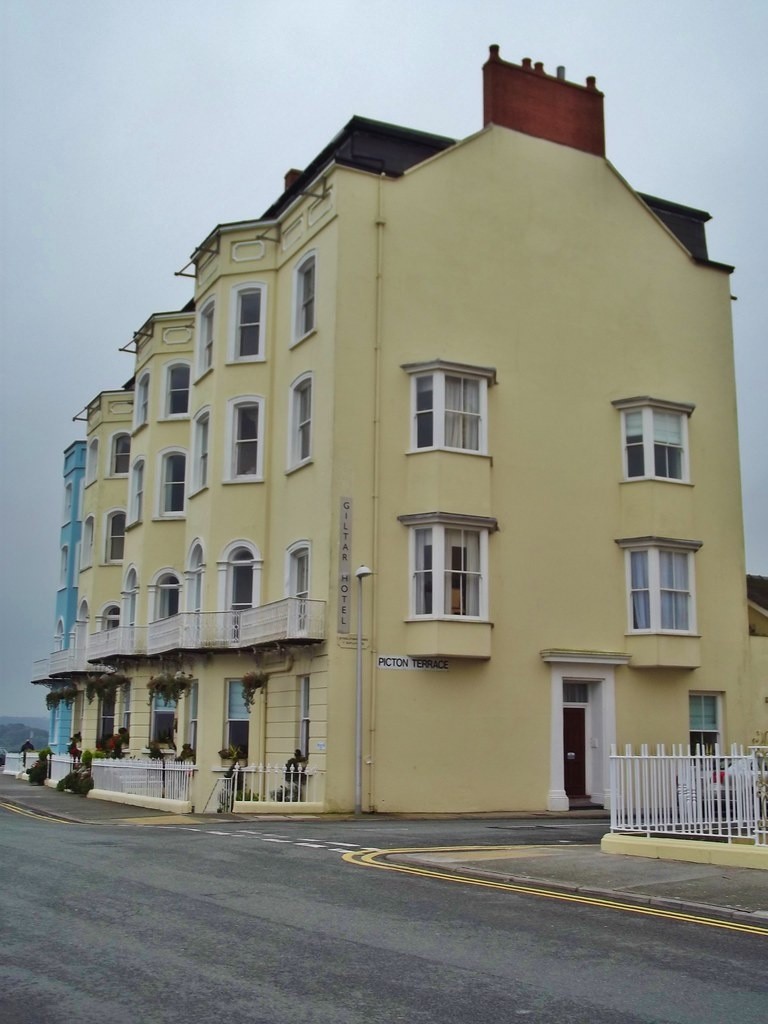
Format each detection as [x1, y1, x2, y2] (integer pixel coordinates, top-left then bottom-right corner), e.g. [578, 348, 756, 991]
[688, 752, 768, 820]
[0, 747, 9, 767]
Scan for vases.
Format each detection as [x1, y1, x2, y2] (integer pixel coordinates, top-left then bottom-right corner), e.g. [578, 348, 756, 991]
[222, 759, 246, 767]
[251, 681, 262, 688]
[57, 693, 65, 699]
[155, 681, 186, 692]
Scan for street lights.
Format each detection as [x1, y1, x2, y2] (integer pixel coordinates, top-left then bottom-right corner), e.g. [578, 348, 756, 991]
[354, 564, 373, 822]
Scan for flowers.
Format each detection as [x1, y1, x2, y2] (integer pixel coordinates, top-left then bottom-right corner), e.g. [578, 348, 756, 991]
[143, 670, 192, 707]
[84, 673, 129, 704]
[239, 671, 268, 711]
[67, 733, 123, 755]
[44, 687, 79, 711]
[220, 746, 246, 760]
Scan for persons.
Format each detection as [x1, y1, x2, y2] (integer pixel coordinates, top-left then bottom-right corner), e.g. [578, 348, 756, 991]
[19, 739, 34, 767]
